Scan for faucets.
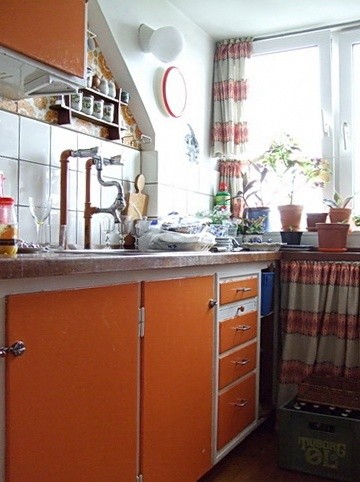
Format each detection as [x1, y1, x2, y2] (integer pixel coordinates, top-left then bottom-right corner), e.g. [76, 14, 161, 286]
[91, 200, 127, 225]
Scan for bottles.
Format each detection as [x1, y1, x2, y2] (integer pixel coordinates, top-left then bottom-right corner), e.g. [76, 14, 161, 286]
[87, 66, 92, 87]
[102, 102, 115, 122]
[109, 81, 116, 98]
[71, 90, 84, 111]
[0, 197, 19, 258]
[92, 73, 101, 92]
[93, 97, 105, 119]
[121, 91, 130, 104]
[214, 184, 230, 211]
[82, 94, 94, 116]
[100, 76, 108, 96]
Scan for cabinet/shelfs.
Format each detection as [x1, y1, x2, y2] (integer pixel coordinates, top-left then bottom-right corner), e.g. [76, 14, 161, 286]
[1, 0, 88, 100]
[50, 86, 129, 140]
[216, 274, 259, 452]
[1, 281, 141, 482]
[142, 275, 218, 481]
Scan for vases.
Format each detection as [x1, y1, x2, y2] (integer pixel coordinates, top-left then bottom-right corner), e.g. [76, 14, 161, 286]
[314, 220, 352, 248]
[306, 212, 329, 233]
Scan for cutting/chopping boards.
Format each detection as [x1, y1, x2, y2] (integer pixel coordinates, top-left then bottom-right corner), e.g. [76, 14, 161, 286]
[123, 174, 149, 220]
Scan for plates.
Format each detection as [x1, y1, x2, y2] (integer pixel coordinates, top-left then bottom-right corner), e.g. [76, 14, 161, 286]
[157, 240, 215, 251]
[280, 245, 313, 251]
[169, 223, 207, 234]
[241, 242, 287, 251]
[318, 247, 348, 252]
[306, 227, 318, 233]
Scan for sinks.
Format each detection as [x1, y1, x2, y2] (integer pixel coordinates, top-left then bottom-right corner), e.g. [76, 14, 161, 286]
[55, 249, 169, 256]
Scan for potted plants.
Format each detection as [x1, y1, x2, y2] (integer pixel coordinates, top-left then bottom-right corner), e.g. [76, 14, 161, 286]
[280, 226, 304, 245]
[240, 214, 268, 244]
[226, 153, 271, 232]
[321, 190, 353, 223]
[257, 132, 336, 231]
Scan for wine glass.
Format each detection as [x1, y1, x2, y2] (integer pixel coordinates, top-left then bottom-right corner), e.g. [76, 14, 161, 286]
[28, 196, 52, 253]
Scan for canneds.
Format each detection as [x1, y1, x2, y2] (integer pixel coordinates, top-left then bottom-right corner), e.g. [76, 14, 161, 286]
[0, 197, 17, 254]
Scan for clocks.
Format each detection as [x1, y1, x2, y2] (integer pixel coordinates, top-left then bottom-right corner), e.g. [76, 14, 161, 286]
[163, 66, 187, 117]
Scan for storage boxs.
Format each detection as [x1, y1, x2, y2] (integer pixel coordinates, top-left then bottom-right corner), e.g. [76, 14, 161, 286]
[276, 394, 359, 481]
